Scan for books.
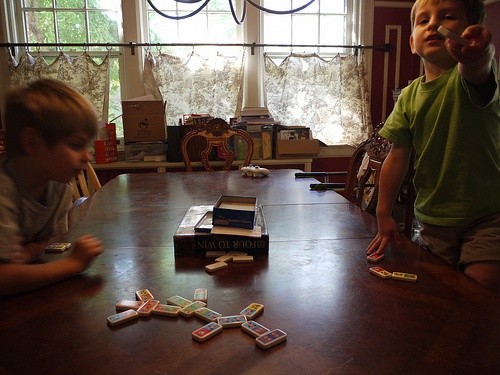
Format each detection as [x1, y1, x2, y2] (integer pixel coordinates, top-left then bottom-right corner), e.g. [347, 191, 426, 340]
[240, 107, 279, 133]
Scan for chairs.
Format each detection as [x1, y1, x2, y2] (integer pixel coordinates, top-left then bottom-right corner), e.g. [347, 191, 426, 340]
[70, 162, 103, 209]
[295, 122, 413, 239]
[181, 118, 254, 172]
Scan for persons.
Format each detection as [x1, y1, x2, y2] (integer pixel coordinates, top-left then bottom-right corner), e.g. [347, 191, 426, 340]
[0, 79, 104, 295]
[366, 0, 500, 292]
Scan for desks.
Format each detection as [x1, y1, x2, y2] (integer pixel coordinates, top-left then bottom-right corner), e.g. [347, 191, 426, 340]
[0, 173, 500, 375]
[93, 158, 312, 173]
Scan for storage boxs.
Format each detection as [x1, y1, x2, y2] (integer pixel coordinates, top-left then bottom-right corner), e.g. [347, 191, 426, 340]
[275, 124, 329, 159]
[173, 204, 269, 258]
[94, 123, 118, 164]
[233, 125, 275, 161]
[121, 95, 169, 141]
[125, 143, 167, 162]
[213, 195, 259, 228]
